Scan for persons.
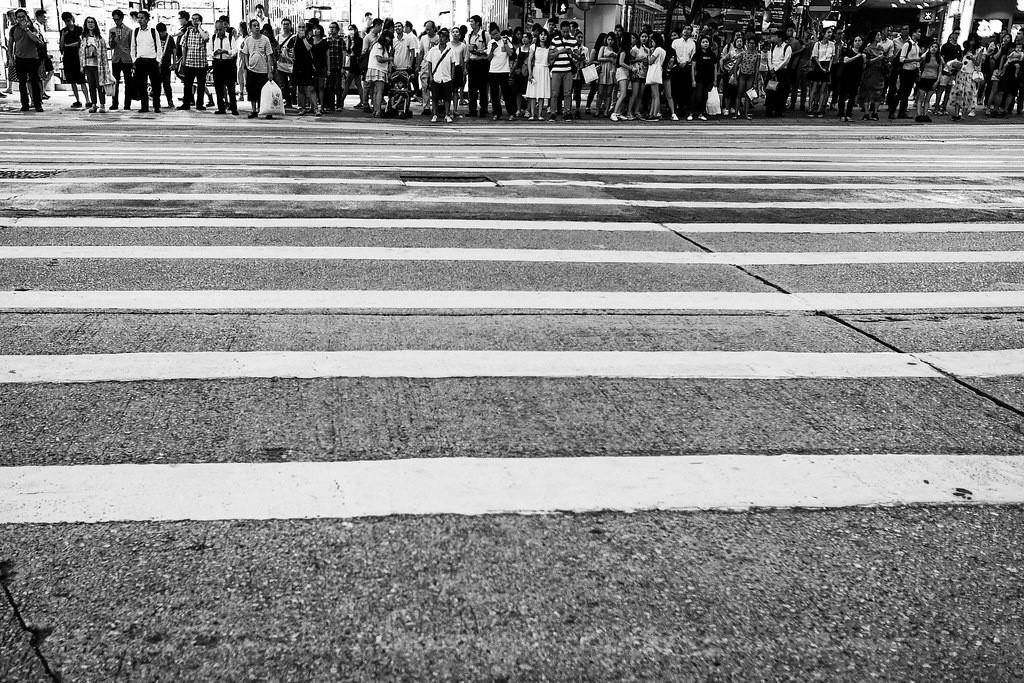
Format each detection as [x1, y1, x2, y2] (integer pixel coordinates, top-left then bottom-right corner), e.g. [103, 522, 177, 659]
[5, 0, 1023, 121]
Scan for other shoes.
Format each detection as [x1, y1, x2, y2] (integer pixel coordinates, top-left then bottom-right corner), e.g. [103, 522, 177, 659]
[21, 93, 1024, 122]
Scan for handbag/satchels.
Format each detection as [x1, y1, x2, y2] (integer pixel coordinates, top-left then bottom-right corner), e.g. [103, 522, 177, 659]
[706, 87, 722, 115]
[277, 61, 294, 73]
[426, 75, 433, 93]
[129, 71, 141, 100]
[645, 49, 663, 85]
[358, 51, 369, 73]
[766, 75, 779, 90]
[259, 81, 286, 115]
[581, 59, 599, 84]
[103, 72, 117, 97]
[972, 64, 984, 82]
[178, 57, 185, 77]
[569, 58, 577, 75]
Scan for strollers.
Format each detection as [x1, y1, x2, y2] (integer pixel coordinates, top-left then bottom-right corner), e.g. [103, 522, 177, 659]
[359, 66, 414, 115]
